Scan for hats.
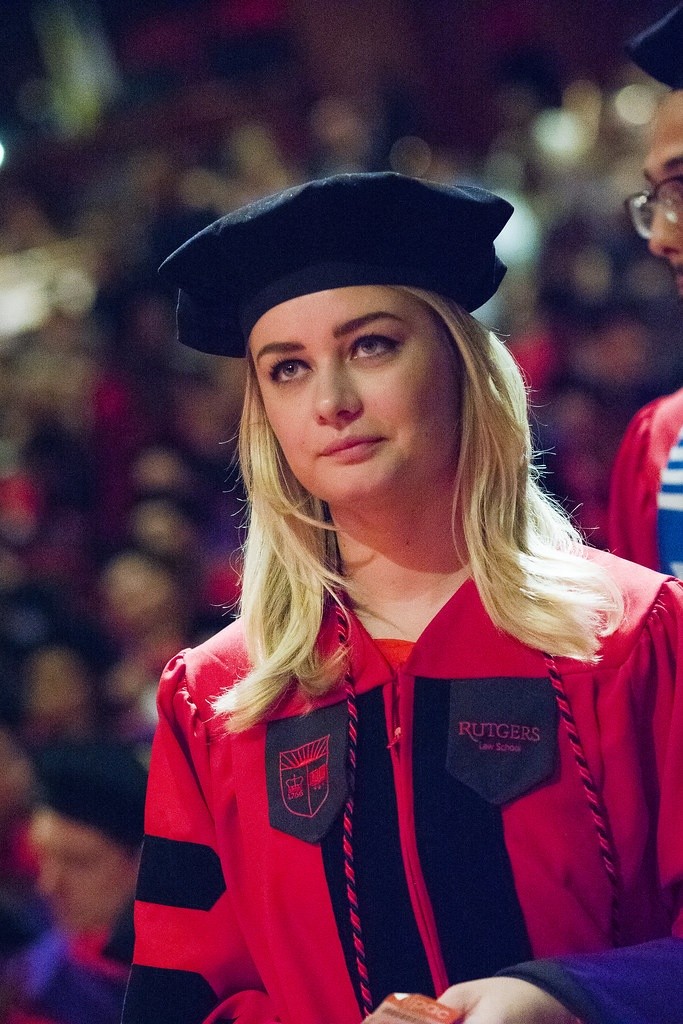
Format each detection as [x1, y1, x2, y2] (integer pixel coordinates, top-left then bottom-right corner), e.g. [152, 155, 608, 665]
[155, 172, 515, 361]
[28, 733, 150, 850]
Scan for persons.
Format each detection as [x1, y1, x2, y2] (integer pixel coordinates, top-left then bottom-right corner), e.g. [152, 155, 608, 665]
[504, 315, 660, 553]
[0, 441, 239, 726]
[121, 171, 683, 1024]
[608, 0, 683, 580]
[0, 728, 149, 1024]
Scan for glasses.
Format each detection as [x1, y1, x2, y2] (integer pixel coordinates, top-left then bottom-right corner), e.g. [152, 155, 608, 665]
[621, 173, 683, 239]
